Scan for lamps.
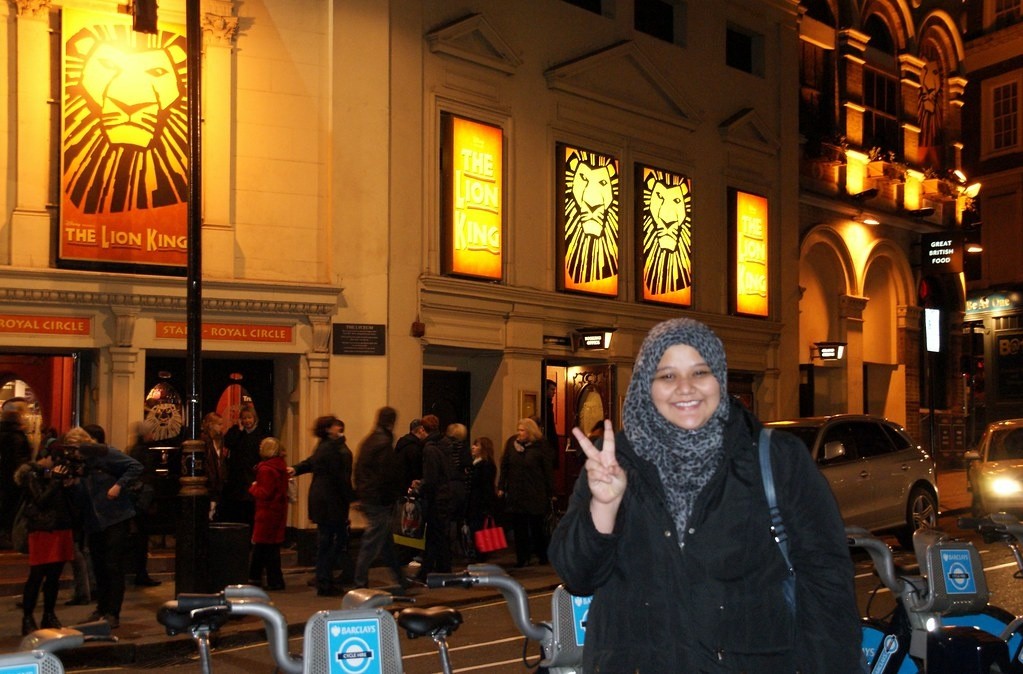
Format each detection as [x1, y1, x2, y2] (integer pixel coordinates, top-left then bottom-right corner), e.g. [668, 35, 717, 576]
[964, 242, 983, 254]
[910, 208, 935, 217]
[810, 341, 849, 363]
[853, 188, 879, 203]
[118, 0, 160, 35]
[575, 325, 617, 351]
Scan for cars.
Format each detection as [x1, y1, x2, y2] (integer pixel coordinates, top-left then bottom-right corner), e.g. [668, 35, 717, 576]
[754, 412, 939, 552]
[962, 418, 1023, 534]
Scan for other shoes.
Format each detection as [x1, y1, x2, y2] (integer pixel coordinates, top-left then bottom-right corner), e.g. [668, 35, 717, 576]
[406, 576, 428, 587]
[511, 563, 533, 570]
[76, 610, 98, 625]
[308, 578, 321, 587]
[64, 598, 89, 605]
[138, 580, 161, 586]
[98, 614, 121, 628]
[22, 616, 38, 636]
[319, 588, 344, 596]
[41, 613, 61, 628]
[264, 583, 284, 591]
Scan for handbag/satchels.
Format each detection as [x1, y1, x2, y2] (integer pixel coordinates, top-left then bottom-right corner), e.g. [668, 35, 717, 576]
[475, 517, 508, 552]
[547, 500, 567, 535]
[286, 476, 299, 503]
[11, 500, 31, 554]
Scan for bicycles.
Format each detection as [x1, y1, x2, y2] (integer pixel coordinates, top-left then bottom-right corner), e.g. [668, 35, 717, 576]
[0, 513, 1023, 674]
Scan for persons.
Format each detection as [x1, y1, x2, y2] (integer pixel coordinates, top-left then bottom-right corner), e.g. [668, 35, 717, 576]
[577, 420, 605, 463]
[199, 407, 293, 592]
[287, 416, 353, 598]
[547, 379, 561, 472]
[352, 407, 415, 589]
[0, 398, 187, 638]
[497, 418, 552, 566]
[395, 415, 497, 589]
[547, 318, 863, 674]
